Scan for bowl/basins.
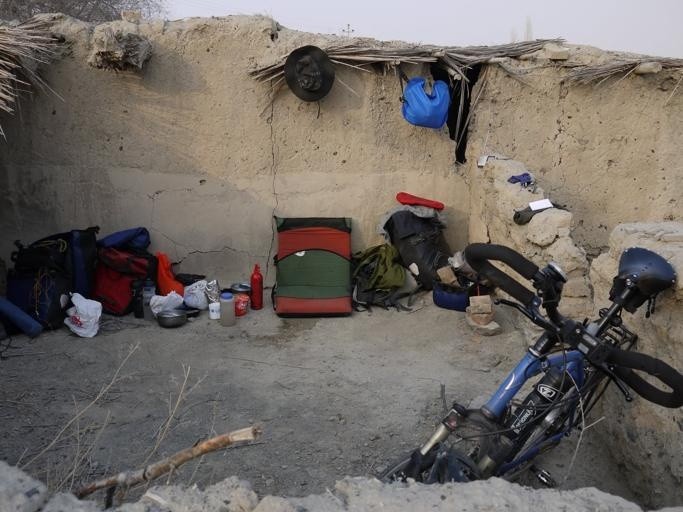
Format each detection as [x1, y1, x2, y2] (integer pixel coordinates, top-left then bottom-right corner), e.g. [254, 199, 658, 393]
[159, 310, 186, 327]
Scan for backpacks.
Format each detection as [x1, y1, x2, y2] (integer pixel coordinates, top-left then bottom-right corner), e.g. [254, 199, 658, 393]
[351, 243, 411, 311]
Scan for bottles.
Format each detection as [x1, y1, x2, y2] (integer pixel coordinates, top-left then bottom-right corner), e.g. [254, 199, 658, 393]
[129, 281, 156, 322]
[220, 294, 234, 325]
[249, 263, 263, 310]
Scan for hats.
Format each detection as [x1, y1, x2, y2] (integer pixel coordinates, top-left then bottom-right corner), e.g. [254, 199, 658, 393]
[284, 46, 335, 102]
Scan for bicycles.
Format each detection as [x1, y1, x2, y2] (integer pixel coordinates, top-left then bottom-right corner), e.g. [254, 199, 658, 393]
[379, 242, 681, 487]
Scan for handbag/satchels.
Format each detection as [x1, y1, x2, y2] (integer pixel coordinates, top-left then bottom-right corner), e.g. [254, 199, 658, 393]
[1, 225, 219, 341]
[384, 210, 493, 312]
[397, 66, 460, 128]
